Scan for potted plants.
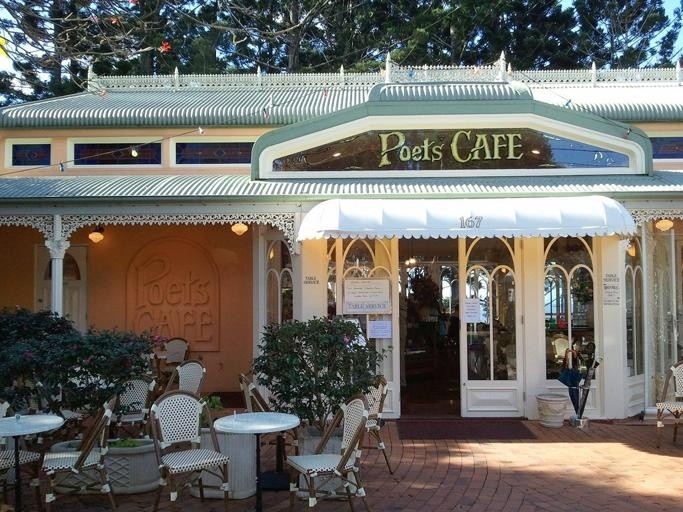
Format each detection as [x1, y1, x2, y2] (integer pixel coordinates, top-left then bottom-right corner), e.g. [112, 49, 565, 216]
[250, 315, 382, 496]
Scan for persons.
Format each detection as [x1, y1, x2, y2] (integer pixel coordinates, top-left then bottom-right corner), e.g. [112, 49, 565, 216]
[450, 304, 459, 318]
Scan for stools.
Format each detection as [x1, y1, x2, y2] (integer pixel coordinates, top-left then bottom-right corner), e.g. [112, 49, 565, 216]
[0, 449, 41, 506]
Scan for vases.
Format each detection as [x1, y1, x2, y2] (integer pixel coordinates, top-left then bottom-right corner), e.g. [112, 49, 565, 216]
[537, 392, 570, 429]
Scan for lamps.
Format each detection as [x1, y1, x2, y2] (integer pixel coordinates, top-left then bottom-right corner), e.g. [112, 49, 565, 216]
[230, 222, 249, 236]
[655, 217, 674, 233]
[88, 224, 106, 244]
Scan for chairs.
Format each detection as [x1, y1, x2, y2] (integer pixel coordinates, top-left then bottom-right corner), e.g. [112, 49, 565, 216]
[149, 390, 230, 510]
[41, 392, 118, 506]
[655, 360, 683, 449]
[284, 394, 371, 510]
[337, 376, 393, 476]
[240, 367, 305, 469]
[40, 338, 208, 440]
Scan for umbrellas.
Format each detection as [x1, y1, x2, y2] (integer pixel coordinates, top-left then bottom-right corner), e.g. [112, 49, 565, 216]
[557, 348, 604, 418]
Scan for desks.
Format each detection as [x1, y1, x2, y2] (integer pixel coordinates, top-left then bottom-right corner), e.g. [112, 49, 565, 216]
[212, 411, 301, 512]
[1, 414, 65, 510]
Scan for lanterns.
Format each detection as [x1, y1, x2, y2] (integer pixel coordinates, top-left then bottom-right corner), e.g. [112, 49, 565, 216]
[627, 243, 638, 257]
[231, 223, 249, 236]
[656, 219, 674, 233]
[87, 227, 105, 244]
